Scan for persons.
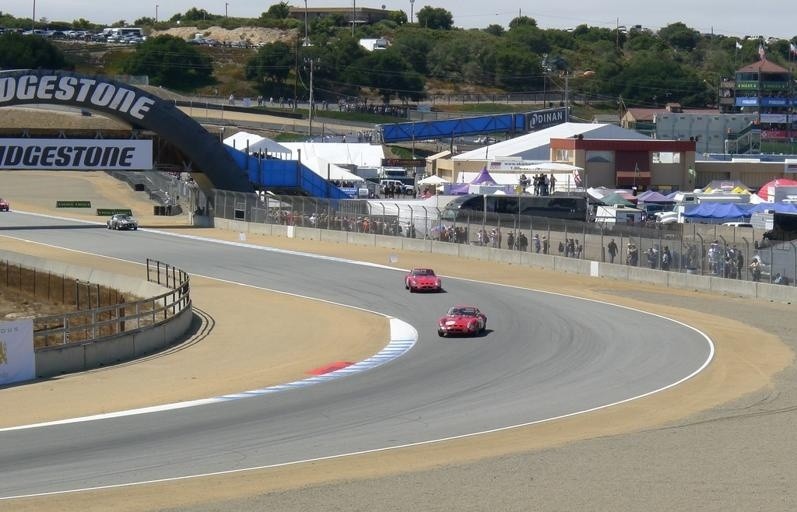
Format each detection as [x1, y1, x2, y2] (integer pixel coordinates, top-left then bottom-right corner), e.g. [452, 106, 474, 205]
[385, 183, 406, 198]
[342, 130, 382, 143]
[195, 206, 205, 216]
[520, 173, 557, 196]
[165, 198, 172, 216]
[228, 94, 407, 118]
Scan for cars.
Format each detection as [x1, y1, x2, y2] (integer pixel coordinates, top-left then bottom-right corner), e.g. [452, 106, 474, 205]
[378, 179, 417, 194]
